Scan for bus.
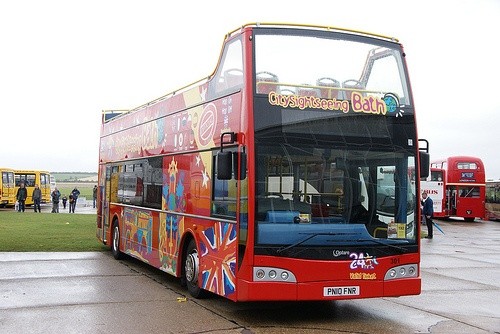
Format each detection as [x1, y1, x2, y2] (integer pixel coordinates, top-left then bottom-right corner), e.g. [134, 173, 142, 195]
[0, 168, 51, 207]
[434, 157, 486, 225]
[418, 168, 445, 216]
[482, 178, 500, 221]
[51, 175, 56, 199]
[95, 23, 429, 302]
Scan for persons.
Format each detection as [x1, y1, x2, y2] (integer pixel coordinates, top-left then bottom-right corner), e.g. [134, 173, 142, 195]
[93, 185, 97, 209]
[71, 186, 80, 206]
[31, 183, 42, 212]
[14, 184, 27, 213]
[69, 193, 75, 213]
[50, 187, 61, 212]
[62, 194, 67, 208]
[421, 192, 435, 239]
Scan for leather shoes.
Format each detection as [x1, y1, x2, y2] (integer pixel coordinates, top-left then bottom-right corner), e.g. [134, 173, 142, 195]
[424, 236, 432, 238]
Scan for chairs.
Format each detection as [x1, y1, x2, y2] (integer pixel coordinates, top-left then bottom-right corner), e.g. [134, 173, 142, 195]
[142, 184, 312, 223]
[217, 69, 368, 100]
